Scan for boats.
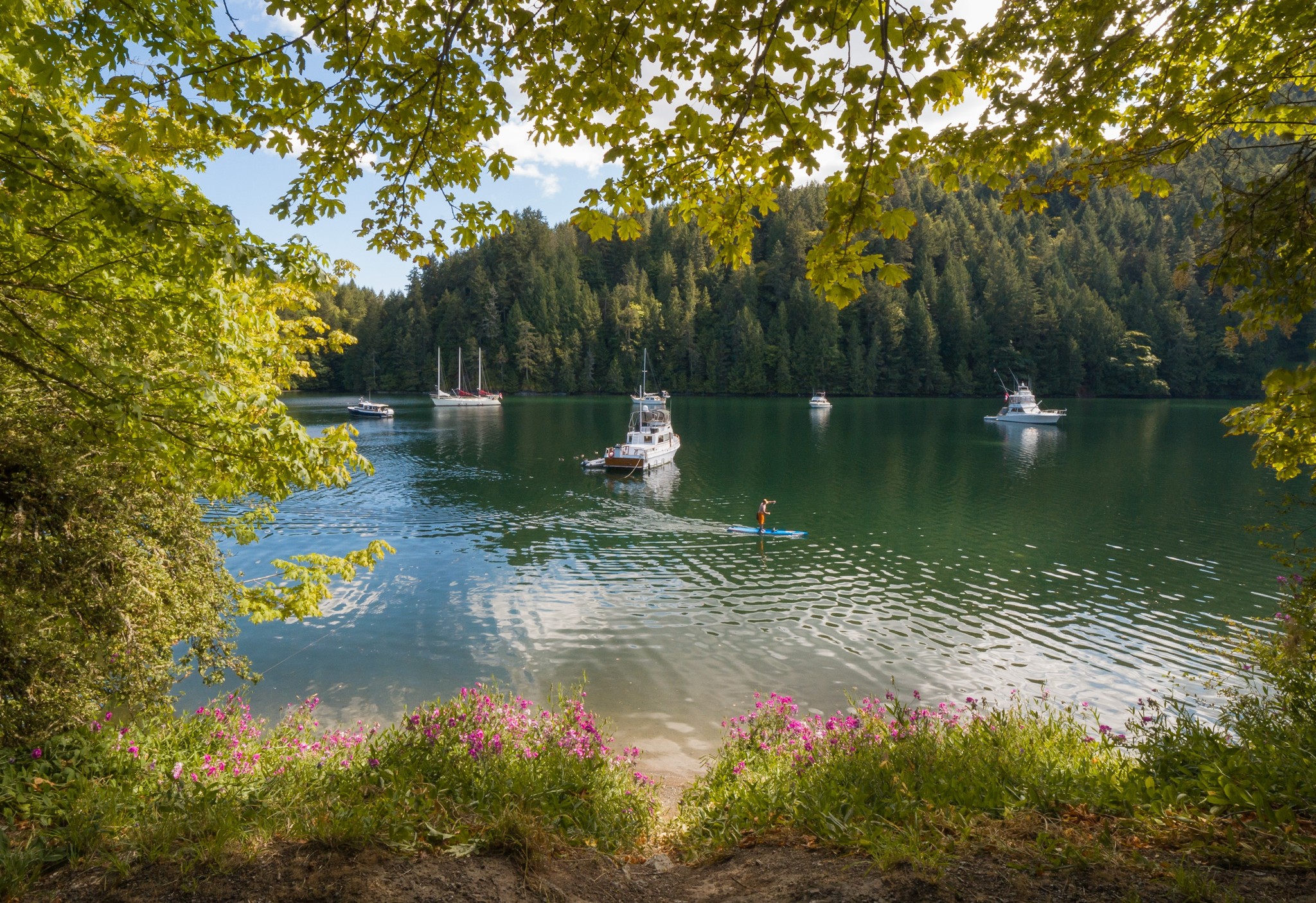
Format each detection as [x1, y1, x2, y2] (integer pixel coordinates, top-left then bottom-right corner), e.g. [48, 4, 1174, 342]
[580, 457, 606, 468]
[808, 388, 833, 408]
[347, 391, 394, 418]
[603, 385, 680, 472]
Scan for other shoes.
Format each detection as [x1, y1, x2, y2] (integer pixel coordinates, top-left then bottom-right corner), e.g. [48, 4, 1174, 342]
[758, 528, 766, 533]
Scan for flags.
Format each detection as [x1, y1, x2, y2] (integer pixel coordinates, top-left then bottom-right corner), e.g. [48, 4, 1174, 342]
[1005, 393, 1008, 402]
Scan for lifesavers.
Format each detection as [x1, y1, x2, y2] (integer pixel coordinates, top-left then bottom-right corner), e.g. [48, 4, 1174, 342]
[608, 451, 614, 458]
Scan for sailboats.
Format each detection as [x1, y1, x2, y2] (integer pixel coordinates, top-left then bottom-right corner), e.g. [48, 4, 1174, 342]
[983, 367, 1067, 426]
[630, 348, 671, 404]
[428, 346, 503, 406]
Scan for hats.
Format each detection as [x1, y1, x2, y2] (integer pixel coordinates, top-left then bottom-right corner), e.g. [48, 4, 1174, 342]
[763, 499, 767, 502]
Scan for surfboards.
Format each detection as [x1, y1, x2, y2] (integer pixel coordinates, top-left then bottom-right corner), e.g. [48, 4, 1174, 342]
[726, 526, 808, 535]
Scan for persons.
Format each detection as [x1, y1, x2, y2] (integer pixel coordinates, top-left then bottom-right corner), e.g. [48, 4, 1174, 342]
[757, 499, 776, 534]
